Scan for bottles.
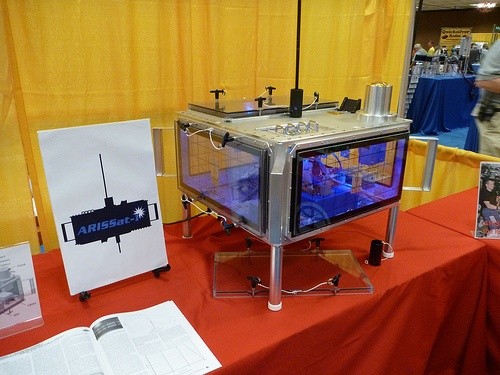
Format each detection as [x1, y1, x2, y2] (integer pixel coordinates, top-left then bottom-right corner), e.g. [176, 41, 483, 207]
[432, 45, 458, 76]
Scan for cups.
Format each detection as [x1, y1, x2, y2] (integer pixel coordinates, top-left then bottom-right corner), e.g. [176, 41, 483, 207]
[472, 64, 480, 74]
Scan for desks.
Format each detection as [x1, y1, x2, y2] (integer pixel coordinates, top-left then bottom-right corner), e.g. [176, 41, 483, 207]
[0, 187, 500, 375]
[409, 73, 480, 136]
